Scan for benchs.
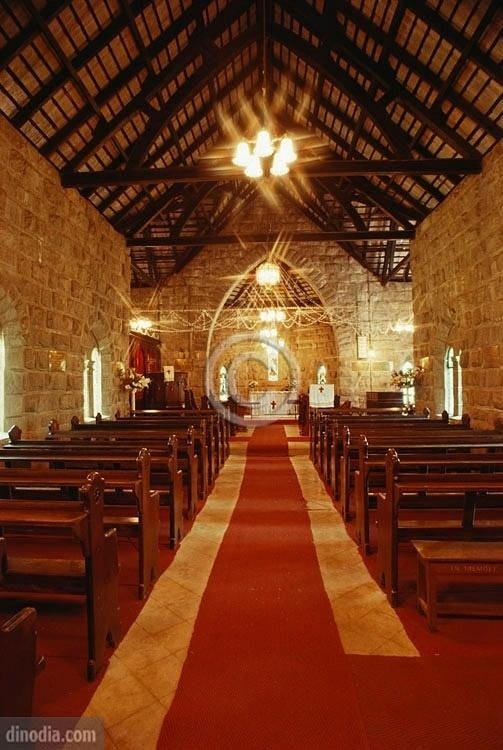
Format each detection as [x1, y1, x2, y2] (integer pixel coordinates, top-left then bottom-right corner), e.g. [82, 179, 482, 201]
[306, 404, 501, 632]
[0, 408, 229, 749]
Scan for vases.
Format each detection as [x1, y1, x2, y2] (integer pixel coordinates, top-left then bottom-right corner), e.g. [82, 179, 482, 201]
[119, 367, 151, 393]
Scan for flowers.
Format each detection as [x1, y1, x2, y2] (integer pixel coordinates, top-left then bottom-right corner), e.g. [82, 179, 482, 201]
[119, 367, 151, 393]
